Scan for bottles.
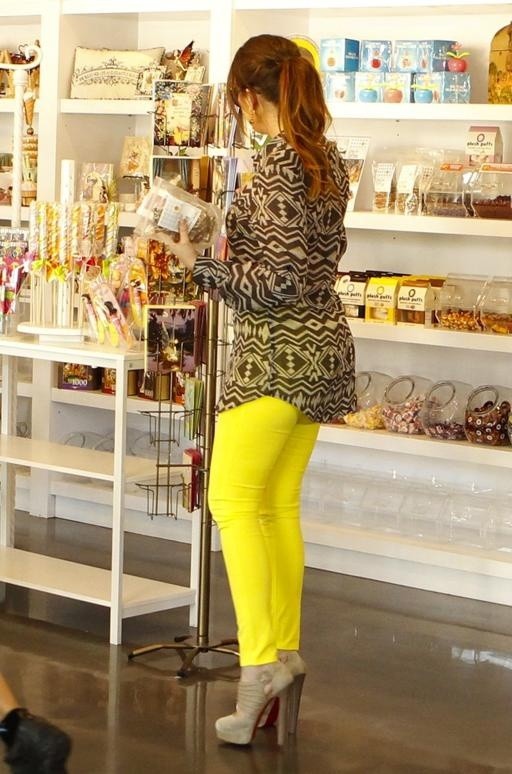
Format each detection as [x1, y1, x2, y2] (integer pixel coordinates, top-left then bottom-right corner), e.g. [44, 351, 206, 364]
[421, 379, 473, 442]
[343, 370, 394, 429]
[462, 384, 512, 446]
[379, 374, 436, 435]
[55, 427, 184, 499]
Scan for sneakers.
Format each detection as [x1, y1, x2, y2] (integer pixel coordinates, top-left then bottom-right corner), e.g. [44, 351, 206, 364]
[3, 712, 70, 774]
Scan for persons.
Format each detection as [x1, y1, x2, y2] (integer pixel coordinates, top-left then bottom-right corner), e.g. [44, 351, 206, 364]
[1, 669, 73, 773]
[163, 32, 361, 748]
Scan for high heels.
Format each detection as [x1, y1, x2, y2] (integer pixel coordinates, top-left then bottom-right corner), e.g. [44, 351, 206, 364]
[214, 650, 307, 746]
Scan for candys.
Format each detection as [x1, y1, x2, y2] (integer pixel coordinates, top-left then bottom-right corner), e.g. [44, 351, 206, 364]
[28, 198, 148, 350]
[343, 391, 511, 446]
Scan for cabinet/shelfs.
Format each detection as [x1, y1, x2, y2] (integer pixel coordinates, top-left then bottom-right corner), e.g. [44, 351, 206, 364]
[0, 341, 207, 645]
[0, 2, 512, 608]
